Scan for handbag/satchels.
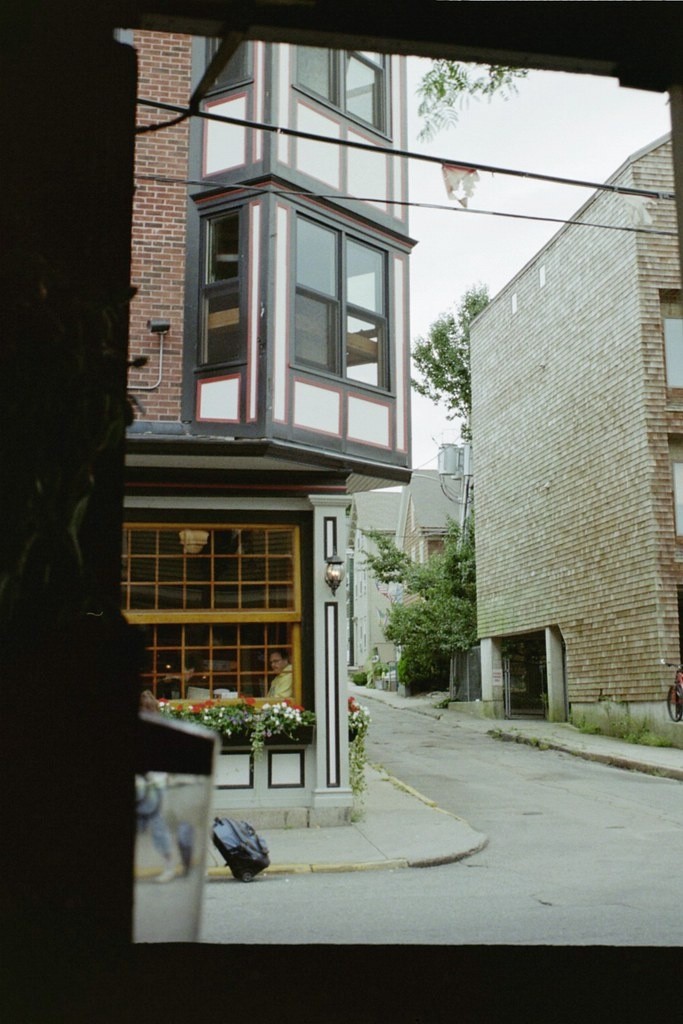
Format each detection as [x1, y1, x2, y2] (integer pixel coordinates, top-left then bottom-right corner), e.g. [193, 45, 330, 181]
[136, 771, 161, 831]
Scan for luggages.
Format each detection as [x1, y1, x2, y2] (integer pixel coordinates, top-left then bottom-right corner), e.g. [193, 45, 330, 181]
[170, 809, 194, 873]
[210, 816, 270, 882]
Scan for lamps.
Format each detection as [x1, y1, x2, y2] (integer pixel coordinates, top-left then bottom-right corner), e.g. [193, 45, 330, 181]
[324, 548, 346, 596]
[179, 528, 209, 555]
[125, 318, 170, 390]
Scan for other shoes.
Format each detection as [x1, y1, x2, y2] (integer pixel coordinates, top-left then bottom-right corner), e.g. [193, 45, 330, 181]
[157, 868, 175, 882]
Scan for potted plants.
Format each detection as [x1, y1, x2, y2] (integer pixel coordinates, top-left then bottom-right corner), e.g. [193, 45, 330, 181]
[351, 672, 367, 686]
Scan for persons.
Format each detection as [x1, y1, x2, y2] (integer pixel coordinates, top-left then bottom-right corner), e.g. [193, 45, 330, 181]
[269, 648, 294, 697]
[155, 672, 175, 701]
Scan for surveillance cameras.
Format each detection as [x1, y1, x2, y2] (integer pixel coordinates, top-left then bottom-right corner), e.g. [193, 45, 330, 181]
[147, 318, 171, 333]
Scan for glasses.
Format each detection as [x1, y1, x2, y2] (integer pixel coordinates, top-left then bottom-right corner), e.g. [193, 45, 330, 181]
[268, 659, 283, 664]
